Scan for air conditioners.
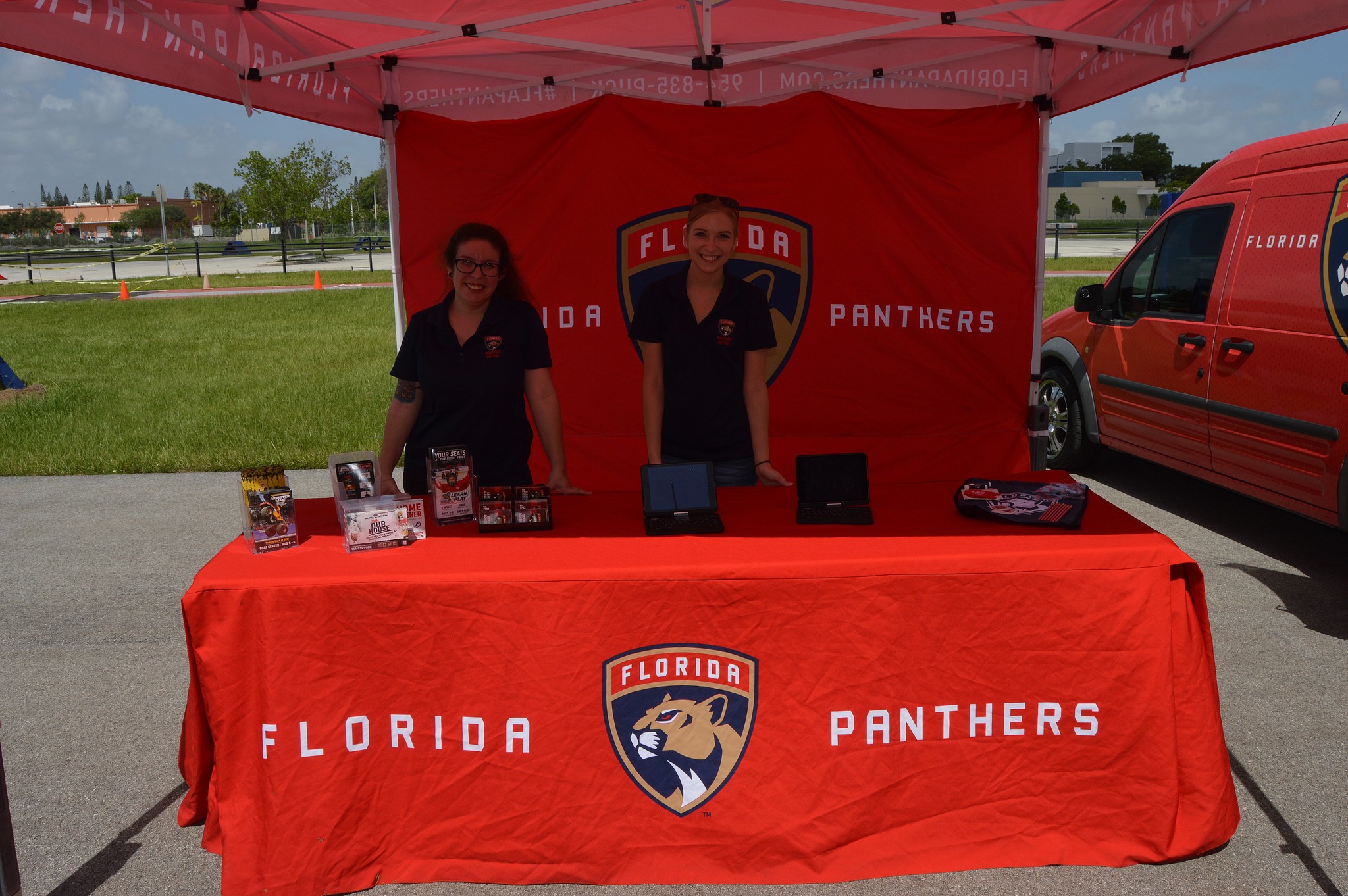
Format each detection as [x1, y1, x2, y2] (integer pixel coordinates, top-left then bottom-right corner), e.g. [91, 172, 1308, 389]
[17, 203, 23, 207]
[106, 200, 112, 204]
[29, 202, 35, 207]
[118, 199, 125, 203]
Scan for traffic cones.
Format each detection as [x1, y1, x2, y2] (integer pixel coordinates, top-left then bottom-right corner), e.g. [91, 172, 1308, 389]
[310, 271, 325, 291]
[116, 280, 132, 301]
[200, 273, 216, 291]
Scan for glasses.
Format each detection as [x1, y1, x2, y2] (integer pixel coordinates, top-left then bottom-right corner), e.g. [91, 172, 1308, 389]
[688, 193, 739, 220]
[452, 258, 501, 276]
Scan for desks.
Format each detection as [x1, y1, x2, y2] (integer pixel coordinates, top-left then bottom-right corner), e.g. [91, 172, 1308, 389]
[191, 469, 1177, 861]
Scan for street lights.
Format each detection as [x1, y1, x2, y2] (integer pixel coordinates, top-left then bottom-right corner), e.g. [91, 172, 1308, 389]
[372, 184, 379, 234]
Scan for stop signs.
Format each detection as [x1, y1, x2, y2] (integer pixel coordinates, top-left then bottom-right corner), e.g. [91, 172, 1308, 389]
[55, 223, 65, 234]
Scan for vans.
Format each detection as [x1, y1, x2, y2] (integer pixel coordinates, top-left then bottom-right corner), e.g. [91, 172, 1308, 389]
[1027, 122, 1347, 553]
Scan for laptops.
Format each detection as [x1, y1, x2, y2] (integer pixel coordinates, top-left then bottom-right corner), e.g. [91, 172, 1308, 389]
[795, 452, 874, 525]
[641, 461, 724, 536]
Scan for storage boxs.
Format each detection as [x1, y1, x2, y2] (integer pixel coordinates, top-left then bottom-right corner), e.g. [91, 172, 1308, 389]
[236, 444, 477, 554]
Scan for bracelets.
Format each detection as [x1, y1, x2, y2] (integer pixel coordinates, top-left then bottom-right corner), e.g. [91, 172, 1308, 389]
[754, 460, 771, 466]
[380, 478, 395, 481]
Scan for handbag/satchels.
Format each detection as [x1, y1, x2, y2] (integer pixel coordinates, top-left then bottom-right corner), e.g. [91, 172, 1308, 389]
[953, 478, 1089, 530]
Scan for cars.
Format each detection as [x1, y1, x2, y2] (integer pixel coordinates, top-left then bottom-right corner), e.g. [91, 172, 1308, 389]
[74, 235, 114, 244]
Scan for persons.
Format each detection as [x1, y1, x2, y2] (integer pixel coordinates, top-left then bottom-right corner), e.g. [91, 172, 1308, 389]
[348, 515, 367, 545]
[379, 223, 592, 498]
[627, 194, 794, 486]
[257, 478, 269, 492]
[432, 472, 471, 493]
